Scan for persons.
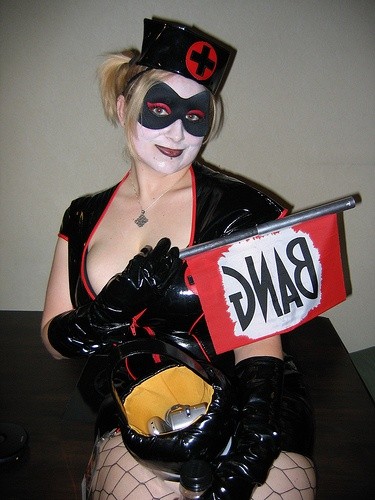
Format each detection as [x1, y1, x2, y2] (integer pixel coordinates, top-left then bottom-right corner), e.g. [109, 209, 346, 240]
[41, 15, 317, 500]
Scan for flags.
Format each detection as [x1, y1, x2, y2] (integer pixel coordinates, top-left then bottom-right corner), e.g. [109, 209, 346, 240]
[179, 196, 356, 355]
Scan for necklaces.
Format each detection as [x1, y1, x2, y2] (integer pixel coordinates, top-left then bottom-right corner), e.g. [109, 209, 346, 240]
[128, 173, 174, 228]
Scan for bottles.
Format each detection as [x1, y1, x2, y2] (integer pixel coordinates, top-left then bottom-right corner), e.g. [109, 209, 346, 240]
[178, 460, 216, 500]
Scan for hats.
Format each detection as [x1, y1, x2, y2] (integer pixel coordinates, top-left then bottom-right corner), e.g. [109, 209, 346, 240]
[135, 16, 238, 96]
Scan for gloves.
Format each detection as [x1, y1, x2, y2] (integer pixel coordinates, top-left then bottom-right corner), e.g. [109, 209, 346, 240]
[47, 238, 186, 360]
[195, 355, 296, 500]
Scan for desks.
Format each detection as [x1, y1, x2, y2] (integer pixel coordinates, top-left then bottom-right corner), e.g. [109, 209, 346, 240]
[0, 310, 375, 500]
